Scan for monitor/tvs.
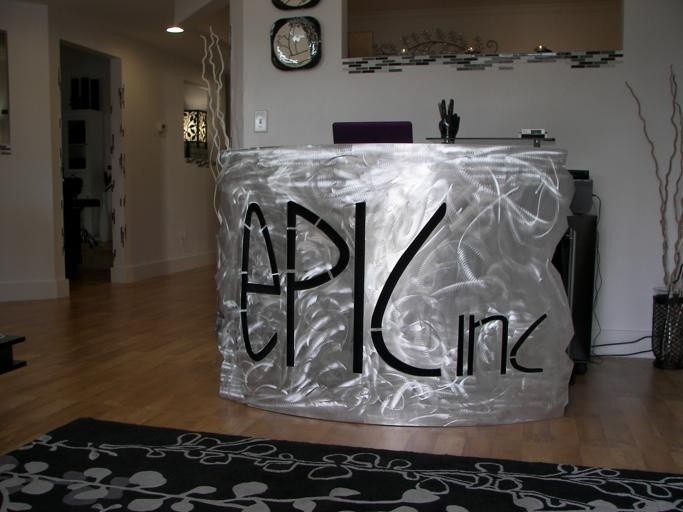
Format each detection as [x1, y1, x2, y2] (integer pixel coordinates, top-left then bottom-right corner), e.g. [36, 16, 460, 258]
[332, 121, 413, 144]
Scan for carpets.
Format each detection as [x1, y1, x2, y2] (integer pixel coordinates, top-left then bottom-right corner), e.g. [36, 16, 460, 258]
[0, 417, 682, 512]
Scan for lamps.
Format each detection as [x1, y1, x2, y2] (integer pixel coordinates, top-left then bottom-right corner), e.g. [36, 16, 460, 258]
[183, 108, 206, 164]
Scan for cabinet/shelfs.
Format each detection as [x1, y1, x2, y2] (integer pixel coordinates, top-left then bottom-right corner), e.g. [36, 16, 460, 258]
[339, 0, 624, 60]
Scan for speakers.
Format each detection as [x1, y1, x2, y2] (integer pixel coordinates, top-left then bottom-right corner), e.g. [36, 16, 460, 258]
[570, 179, 593, 215]
